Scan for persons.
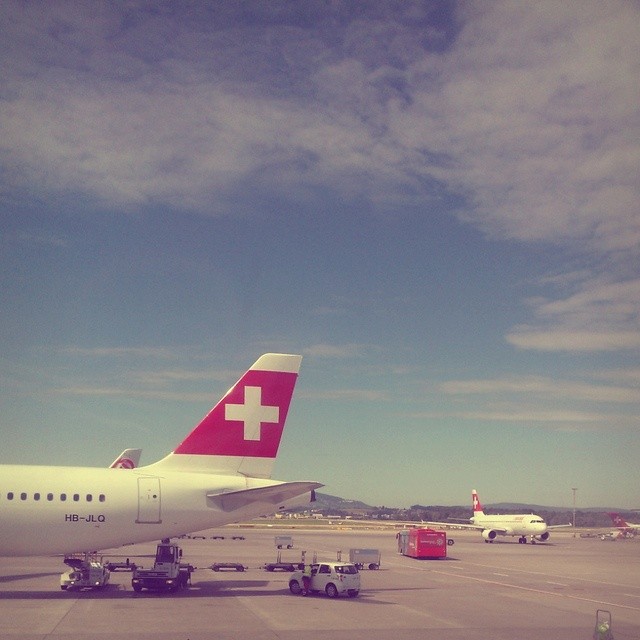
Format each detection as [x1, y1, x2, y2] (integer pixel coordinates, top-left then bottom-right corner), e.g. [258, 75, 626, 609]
[302, 560, 312, 594]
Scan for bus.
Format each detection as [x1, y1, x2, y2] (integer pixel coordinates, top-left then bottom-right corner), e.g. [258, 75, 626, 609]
[396, 530, 447, 558]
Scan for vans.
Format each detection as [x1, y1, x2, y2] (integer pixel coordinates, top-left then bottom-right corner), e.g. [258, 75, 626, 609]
[289, 561, 360, 598]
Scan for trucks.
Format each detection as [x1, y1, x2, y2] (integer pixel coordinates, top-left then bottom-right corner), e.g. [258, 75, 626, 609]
[132, 542, 192, 594]
[58, 554, 110, 591]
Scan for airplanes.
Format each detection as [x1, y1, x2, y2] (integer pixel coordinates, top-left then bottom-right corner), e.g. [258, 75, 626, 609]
[0, 351, 325, 558]
[418, 490, 573, 544]
[608, 511, 640, 537]
